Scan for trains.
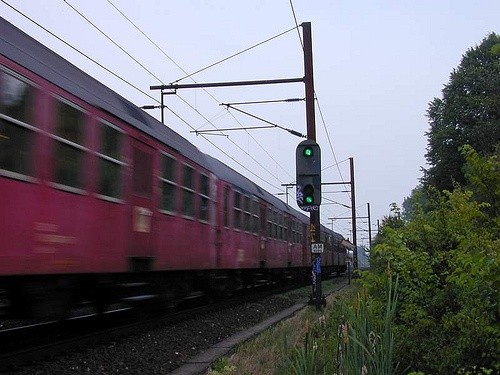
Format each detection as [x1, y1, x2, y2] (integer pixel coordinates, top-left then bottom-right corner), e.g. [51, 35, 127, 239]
[0, 16, 349, 326]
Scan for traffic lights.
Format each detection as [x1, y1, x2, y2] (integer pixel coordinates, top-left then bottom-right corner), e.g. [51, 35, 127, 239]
[295, 139, 322, 213]
